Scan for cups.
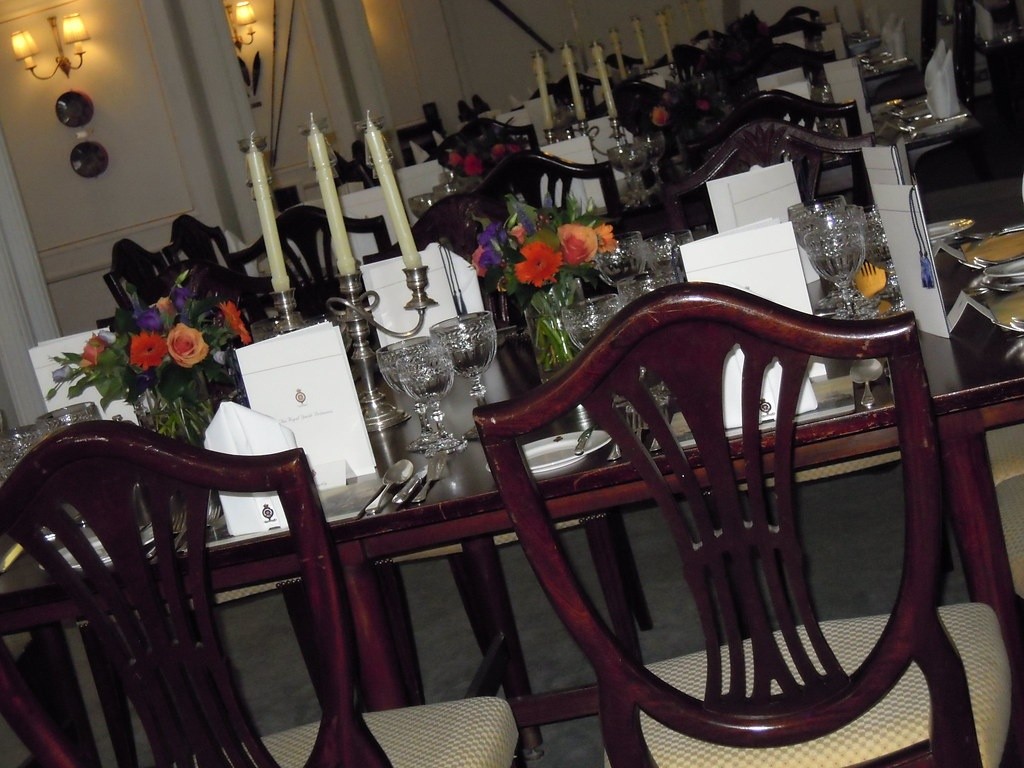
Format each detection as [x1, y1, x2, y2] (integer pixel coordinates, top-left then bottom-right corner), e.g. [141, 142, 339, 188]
[0, 424, 59, 479]
[560, 230, 694, 411]
[36, 402, 101, 432]
[407, 171, 460, 220]
[811, 85, 844, 140]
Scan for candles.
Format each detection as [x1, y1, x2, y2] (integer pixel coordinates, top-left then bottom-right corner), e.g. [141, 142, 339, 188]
[308, 112, 358, 276]
[246, 131, 290, 291]
[365, 109, 421, 266]
[655, 9, 675, 63]
[588, 40, 617, 119]
[678, 0, 696, 40]
[630, 15, 651, 70]
[607, 28, 629, 80]
[698, 0, 713, 35]
[528, 47, 556, 130]
[561, 39, 587, 120]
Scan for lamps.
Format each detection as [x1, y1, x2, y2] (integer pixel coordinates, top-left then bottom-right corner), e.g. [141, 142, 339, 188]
[10, 13, 90, 80]
[226, 2, 257, 50]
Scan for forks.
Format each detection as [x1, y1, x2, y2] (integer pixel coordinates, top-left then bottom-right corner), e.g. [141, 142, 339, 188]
[173, 506, 221, 549]
[412, 459, 445, 501]
[144, 511, 186, 558]
[633, 412, 649, 442]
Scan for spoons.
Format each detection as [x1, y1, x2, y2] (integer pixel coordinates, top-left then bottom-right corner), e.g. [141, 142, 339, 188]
[849, 357, 883, 406]
[364, 459, 413, 512]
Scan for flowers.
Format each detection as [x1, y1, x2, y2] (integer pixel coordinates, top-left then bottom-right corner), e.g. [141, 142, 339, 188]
[438, 115, 524, 179]
[46, 264, 254, 448]
[717, 11, 771, 45]
[646, 69, 731, 127]
[467, 187, 623, 365]
[699, 32, 755, 76]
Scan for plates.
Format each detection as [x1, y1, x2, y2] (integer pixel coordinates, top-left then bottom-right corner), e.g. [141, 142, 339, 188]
[486, 429, 612, 474]
[40, 526, 155, 568]
[984, 258, 1024, 278]
[926, 219, 973, 240]
[848, 37, 881, 54]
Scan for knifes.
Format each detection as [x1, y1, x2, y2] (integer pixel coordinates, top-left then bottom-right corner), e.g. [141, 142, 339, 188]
[573, 424, 596, 454]
[391, 465, 429, 504]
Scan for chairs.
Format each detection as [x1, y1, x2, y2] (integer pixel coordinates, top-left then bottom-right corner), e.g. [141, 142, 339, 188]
[0, 0, 1024, 768]
[2, 4, 1016, 768]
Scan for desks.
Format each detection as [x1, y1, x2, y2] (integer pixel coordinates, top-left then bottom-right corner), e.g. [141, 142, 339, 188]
[628, 93, 982, 237]
[0, 181, 1023, 768]
[812, 50, 918, 86]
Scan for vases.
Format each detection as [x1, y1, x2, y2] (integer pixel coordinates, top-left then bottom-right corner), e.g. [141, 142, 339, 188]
[674, 115, 725, 172]
[131, 370, 226, 523]
[522, 272, 587, 386]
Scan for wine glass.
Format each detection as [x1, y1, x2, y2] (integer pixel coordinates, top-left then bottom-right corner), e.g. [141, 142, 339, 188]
[429, 310, 497, 440]
[788, 194, 906, 321]
[396, 346, 467, 457]
[376, 336, 452, 452]
[608, 131, 666, 207]
